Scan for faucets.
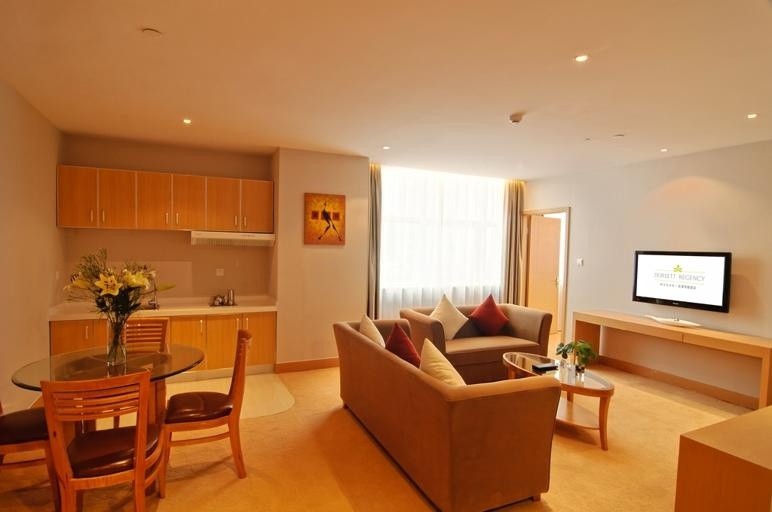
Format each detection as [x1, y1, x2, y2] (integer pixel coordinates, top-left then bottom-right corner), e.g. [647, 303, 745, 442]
[148, 295, 158, 309]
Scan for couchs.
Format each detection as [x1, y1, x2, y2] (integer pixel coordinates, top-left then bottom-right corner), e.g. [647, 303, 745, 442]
[398, 301, 553, 385]
[331, 320, 562, 511]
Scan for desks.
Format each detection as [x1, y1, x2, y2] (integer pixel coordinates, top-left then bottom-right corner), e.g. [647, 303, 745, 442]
[11, 343, 205, 496]
[501, 349, 615, 451]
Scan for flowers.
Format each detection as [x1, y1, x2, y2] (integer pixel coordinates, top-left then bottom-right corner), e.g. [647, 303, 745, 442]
[64, 250, 163, 362]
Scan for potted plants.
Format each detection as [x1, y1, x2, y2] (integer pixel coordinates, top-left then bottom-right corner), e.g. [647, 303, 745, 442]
[555, 339, 597, 376]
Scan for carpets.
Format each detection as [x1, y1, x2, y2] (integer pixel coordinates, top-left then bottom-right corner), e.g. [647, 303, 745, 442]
[93, 369, 296, 432]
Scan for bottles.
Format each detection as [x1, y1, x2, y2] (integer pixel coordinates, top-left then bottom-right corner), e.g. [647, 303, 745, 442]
[227, 289, 235, 304]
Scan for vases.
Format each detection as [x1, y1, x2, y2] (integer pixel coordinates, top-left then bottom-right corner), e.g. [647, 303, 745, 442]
[102, 308, 130, 367]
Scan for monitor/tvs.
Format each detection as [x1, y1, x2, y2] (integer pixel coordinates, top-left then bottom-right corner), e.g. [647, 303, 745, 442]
[632, 250, 731, 314]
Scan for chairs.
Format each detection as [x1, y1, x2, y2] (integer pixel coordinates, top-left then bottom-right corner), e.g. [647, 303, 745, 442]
[40, 368, 168, 511]
[110, 315, 170, 427]
[1, 394, 62, 511]
[155, 328, 255, 483]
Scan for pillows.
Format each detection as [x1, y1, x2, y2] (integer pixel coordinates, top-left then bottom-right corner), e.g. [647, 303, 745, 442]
[428, 293, 469, 343]
[359, 314, 387, 350]
[466, 292, 511, 336]
[384, 320, 423, 368]
[417, 336, 468, 387]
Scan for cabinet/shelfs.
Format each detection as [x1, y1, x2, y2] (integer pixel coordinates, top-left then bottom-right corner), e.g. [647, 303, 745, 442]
[208, 175, 274, 233]
[206, 312, 277, 370]
[50, 319, 132, 375]
[674, 401, 771, 511]
[569, 307, 772, 413]
[135, 170, 208, 229]
[129, 314, 206, 370]
[56, 164, 137, 228]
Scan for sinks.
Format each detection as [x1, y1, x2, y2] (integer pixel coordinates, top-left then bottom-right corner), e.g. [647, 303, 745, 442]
[134, 304, 160, 310]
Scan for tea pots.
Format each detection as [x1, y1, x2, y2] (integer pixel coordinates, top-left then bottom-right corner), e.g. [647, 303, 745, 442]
[211, 294, 226, 306]
